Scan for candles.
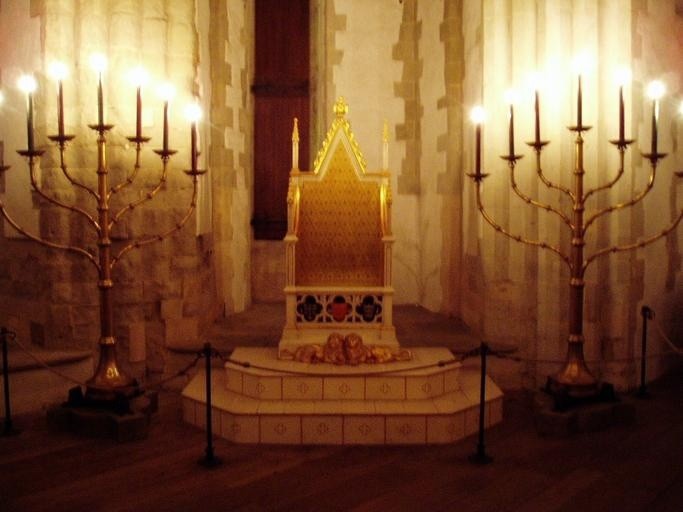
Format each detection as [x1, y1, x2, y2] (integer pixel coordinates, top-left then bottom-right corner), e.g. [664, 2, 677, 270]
[0, 47, 208, 172]
[472, 46, 683, 177]
